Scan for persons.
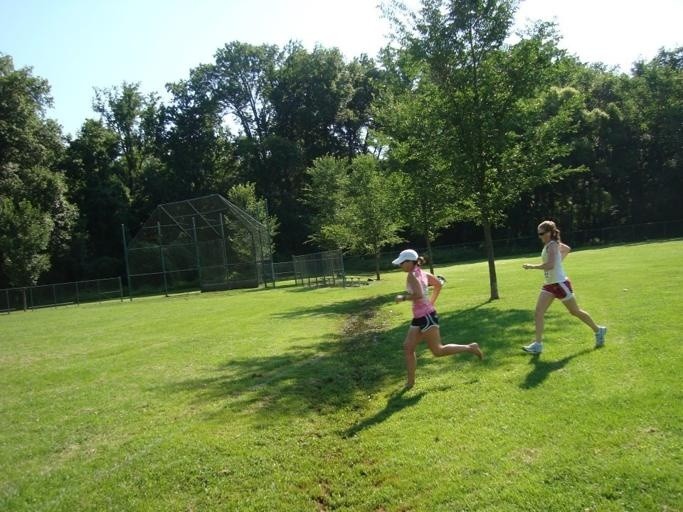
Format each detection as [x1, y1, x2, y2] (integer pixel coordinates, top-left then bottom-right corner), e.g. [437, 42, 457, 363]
[391, 249, 483, 389]
[521, 220, 608, 355]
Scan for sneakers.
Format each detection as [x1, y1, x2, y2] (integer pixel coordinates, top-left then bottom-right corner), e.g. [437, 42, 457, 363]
[596, 327, 606, 346]
[522, 341, 543, 353]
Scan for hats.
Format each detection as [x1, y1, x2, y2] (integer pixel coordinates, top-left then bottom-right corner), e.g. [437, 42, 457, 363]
[392, 249, 418, 265]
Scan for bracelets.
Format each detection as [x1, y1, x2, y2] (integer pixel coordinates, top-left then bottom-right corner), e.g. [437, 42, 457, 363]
[402, 295, 406, 300]
